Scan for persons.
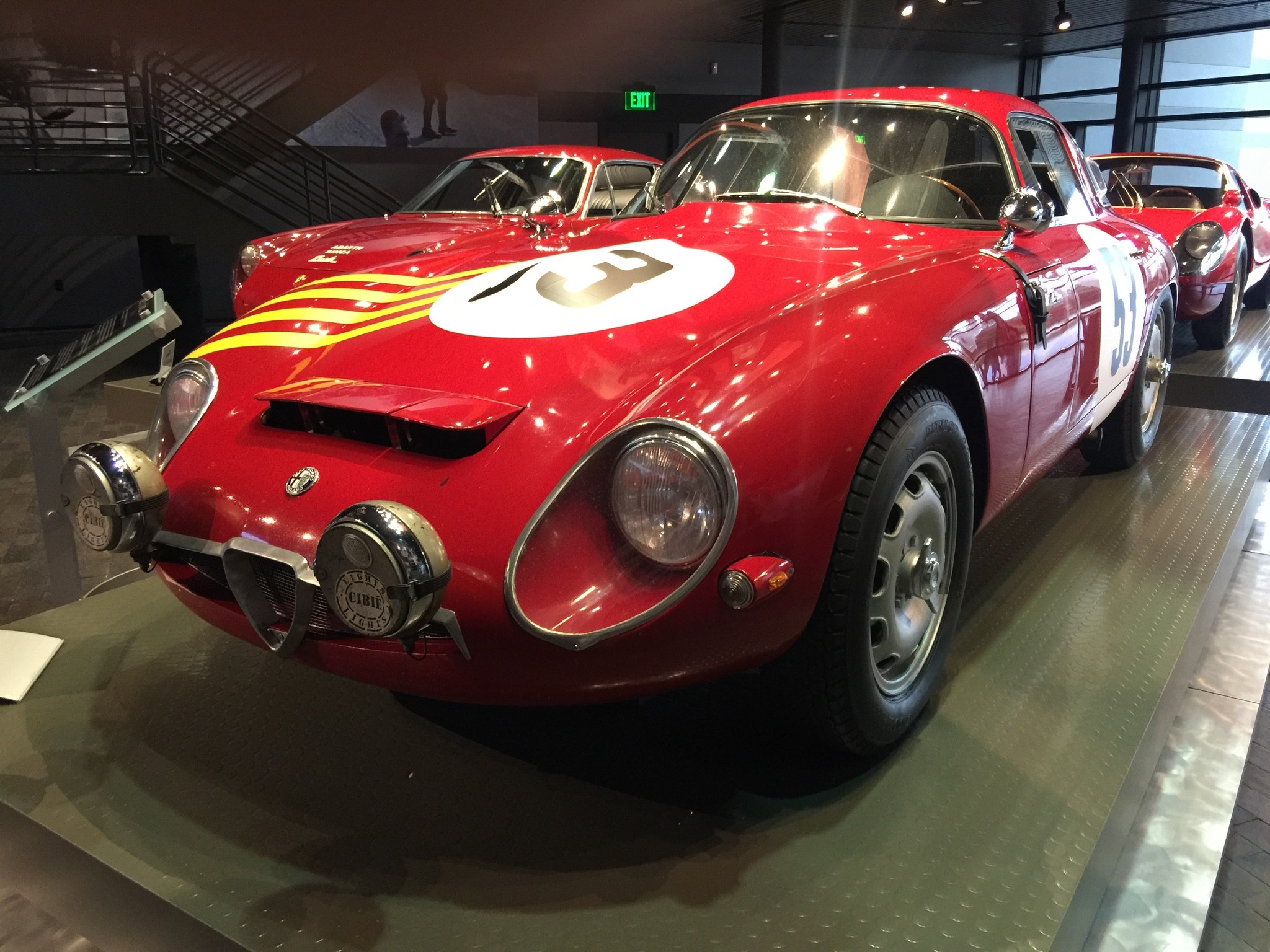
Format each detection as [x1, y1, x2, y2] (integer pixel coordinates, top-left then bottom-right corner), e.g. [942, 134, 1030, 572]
[381, 109, 410, 148]
[414, 49, 457, 141]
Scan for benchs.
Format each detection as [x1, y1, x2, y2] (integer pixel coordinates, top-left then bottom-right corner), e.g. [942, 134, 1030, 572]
[1104, 185, 1224, 210]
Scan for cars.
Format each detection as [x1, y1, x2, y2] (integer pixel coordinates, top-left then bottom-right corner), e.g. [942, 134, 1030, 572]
[1090, 152, 1270, 348]
[69, 86, 1178, 757]
[230, 143, 663, 323]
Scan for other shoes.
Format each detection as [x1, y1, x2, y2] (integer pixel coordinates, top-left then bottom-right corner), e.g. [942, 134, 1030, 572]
[438, 125, 457, 134]
[422, 128, 442, 140]
